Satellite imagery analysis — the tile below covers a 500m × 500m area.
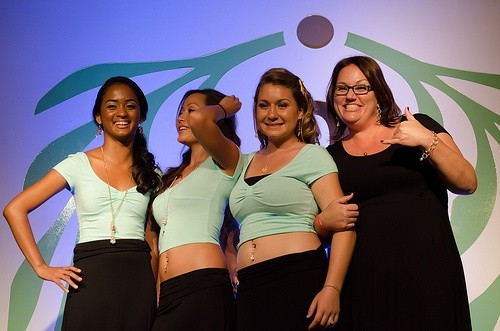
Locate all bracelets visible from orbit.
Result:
[218,104,227,118]
[316,214,327,233]
[323,283,341,294]
[420,131,439,161]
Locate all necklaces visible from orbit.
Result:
[262,138,298,174]
[100,146,132,244]
[162,155,205,236]
[350,127,379,156]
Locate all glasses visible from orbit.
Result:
[333,84,373,96]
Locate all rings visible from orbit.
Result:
[328,318,333,321]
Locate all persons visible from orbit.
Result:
[145,89,243,331]
[3,76,162,331]
[225,67,359,331]
[313,56,477,331]
